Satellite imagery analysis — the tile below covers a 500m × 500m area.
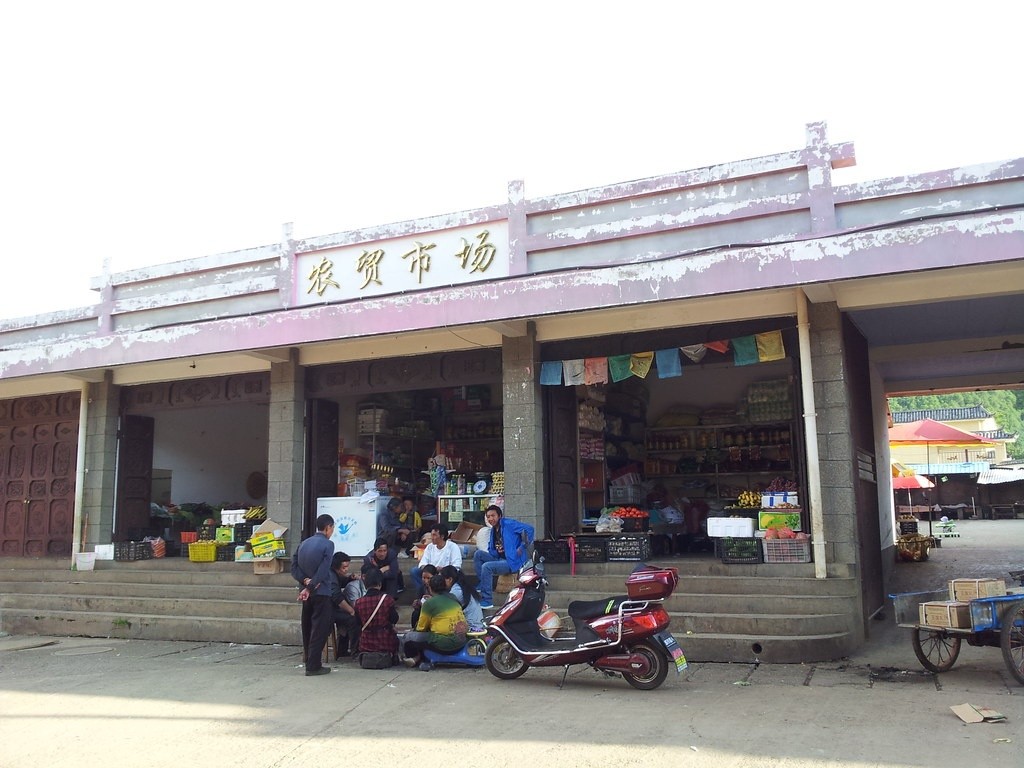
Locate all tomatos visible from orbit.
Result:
[609,506,649,529]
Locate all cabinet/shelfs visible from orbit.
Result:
[639,420,798,501]
[550,383,646,536]
[441,409,503,472]
[354,403,436,507]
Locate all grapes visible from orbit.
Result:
[764,477,797,491]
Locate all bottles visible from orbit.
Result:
[449,474,466,494]
[698,428,714,448]
[466,483,474,494]
[647,429,691,450]
[718,427,790,446]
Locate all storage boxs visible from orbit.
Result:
[896,505,929,512]
[918,578,1024,628]
[95,502,290,574]
[624,566,679,601]
[533,459,677,563]
[337,409,435,497]
[495,572,518,593]
[409,520,485,560]
[707,491,812,564]
[900,522,918,534]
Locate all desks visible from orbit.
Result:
[932,505,983,520]
[986,504,1024,519]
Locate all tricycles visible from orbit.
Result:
[888,569,1024,685]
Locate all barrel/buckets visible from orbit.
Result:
[75,553,95,571]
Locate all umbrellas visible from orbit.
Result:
[893,475,935,516]
[888,418,996,536]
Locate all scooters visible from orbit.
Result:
[480,549,690,691]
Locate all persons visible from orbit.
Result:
[411,565,439,629]
[377,497,414,559]
[399,496,422,531]
[473,505,534,609]
[410,524,463,591]
[364,538,399,594]
[343,563,375,609]
[441,565,484,631]
[290,514,335,676]
[355,567,401,665]
[401,575,468,668]
[330,552,361,662]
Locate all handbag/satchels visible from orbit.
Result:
[595,513,624,534]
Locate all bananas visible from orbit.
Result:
[737,490,761,508]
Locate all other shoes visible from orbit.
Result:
[401,654,416,667]
[396,552,409,559]
[337,651,351,658]
[353,656,359,663]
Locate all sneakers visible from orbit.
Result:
[479,602,493,609]
[475,586,482,592]
[306,667,331,676]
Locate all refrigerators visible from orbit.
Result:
[315,497,395,557]
